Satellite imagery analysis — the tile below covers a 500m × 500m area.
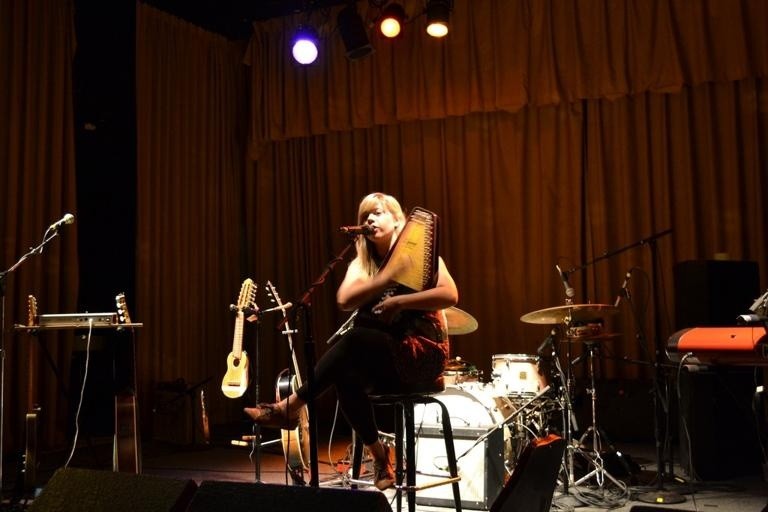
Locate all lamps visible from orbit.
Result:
[289,0,454,67]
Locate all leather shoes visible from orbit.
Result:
[371,442,395,491]
[242,402,300,431]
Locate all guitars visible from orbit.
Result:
[221,278,260,398]
[264,279,311,472]
[113,293,143,473]
[22,296,41,500]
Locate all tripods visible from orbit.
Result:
[561,335,632,496]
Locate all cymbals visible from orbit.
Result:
[521,304,619,325]
[445,306,478,336]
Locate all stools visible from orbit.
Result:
[351,394,461,512]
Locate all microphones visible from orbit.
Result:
[615,271,631,308]
[50,214,74,229]
[336,225,374,234]
[556,265,575,297]
[445,467,460,472]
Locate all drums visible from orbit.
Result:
[399,382,524,512]
[492,355,547,400]
[443,359,479,385]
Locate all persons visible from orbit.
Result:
[239,192,459,492]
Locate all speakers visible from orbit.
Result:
[29,467,197,512]
[413,423,503,510]
[184,480,394,512]
